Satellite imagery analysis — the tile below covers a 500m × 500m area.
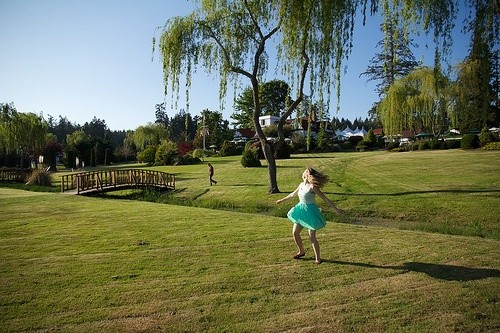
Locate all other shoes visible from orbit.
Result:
[315,259,323,264]
[294,252,306,259]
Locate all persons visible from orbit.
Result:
[276,167,345,264]
[207,162,218,186]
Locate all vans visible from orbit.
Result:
[382,134,410,146]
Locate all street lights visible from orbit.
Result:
[37,155,44,175]
